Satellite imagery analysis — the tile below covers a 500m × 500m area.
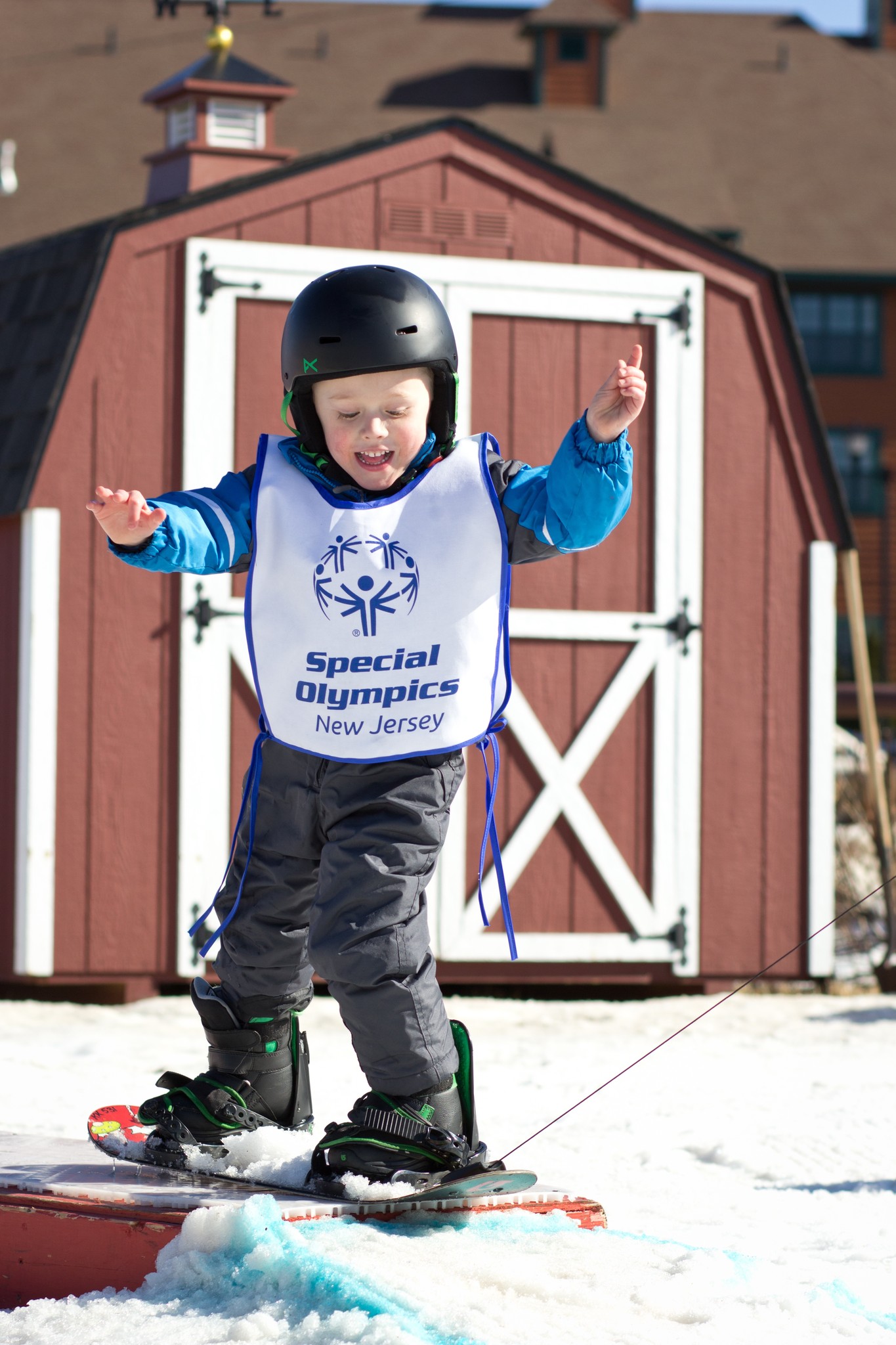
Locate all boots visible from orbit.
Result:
[156,977,313,1139]
[323,1020,477,1175]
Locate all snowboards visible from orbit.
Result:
[86,1106,538,1209]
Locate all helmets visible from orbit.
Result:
[281,264,458,469]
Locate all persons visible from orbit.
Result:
[83,263,652,1190]
